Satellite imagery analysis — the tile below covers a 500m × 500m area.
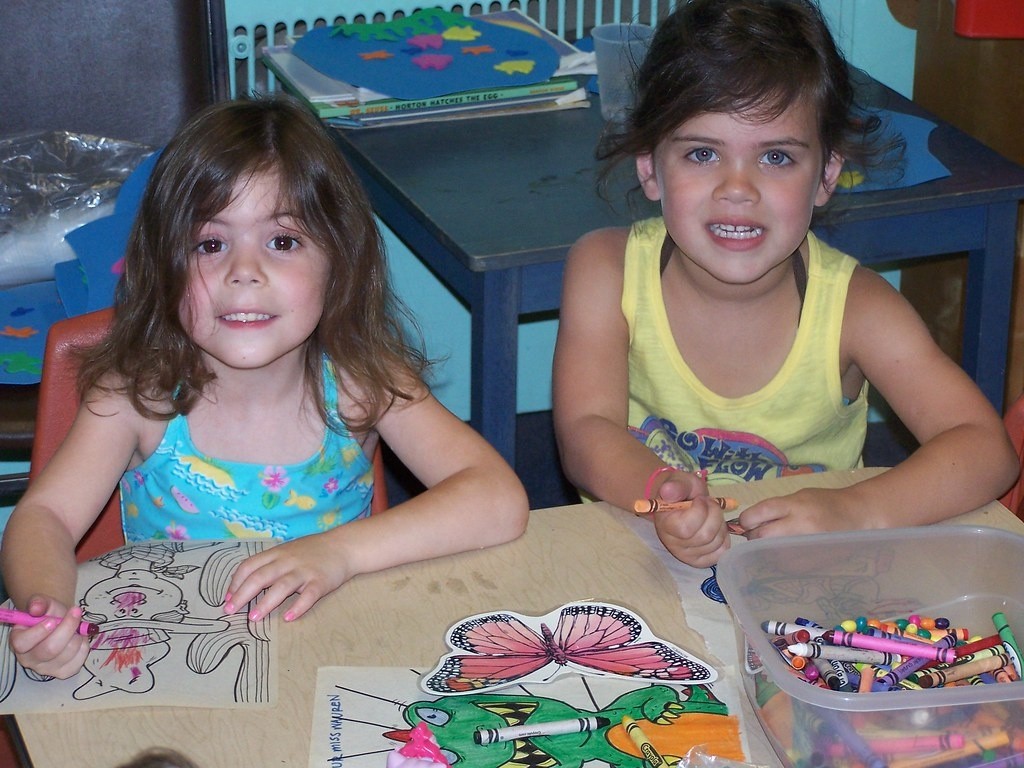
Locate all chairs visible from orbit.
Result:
[26,304,390,567]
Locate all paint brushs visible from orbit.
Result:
[634,496,739,513]
[757,609,1024,767]
[621,714,673,768]
[473,714,611,746]
[0,607,100,637]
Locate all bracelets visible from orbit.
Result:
[643,465,708,518]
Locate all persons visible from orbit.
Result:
[0,88,528,680]
[549,0,1024,570]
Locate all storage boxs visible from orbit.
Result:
[716,526,1023,768]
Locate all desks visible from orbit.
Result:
[1,470,1023,768]
[291,37,1024,504]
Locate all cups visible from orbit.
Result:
[590,22,656,123]
